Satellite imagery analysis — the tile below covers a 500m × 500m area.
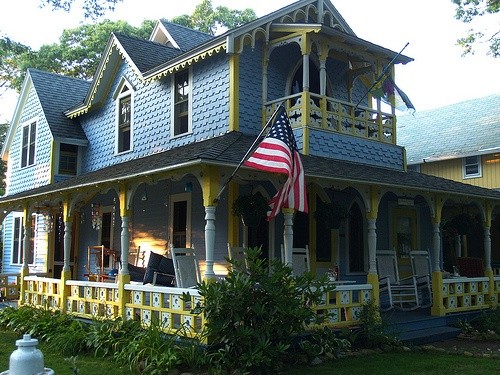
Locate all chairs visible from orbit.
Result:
[226,243,250,280]
[409,248,433,309]
[169,242,204,292]
[280,245,312,280]
[375,247,423,312]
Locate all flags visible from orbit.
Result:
[243,105,309,222]
